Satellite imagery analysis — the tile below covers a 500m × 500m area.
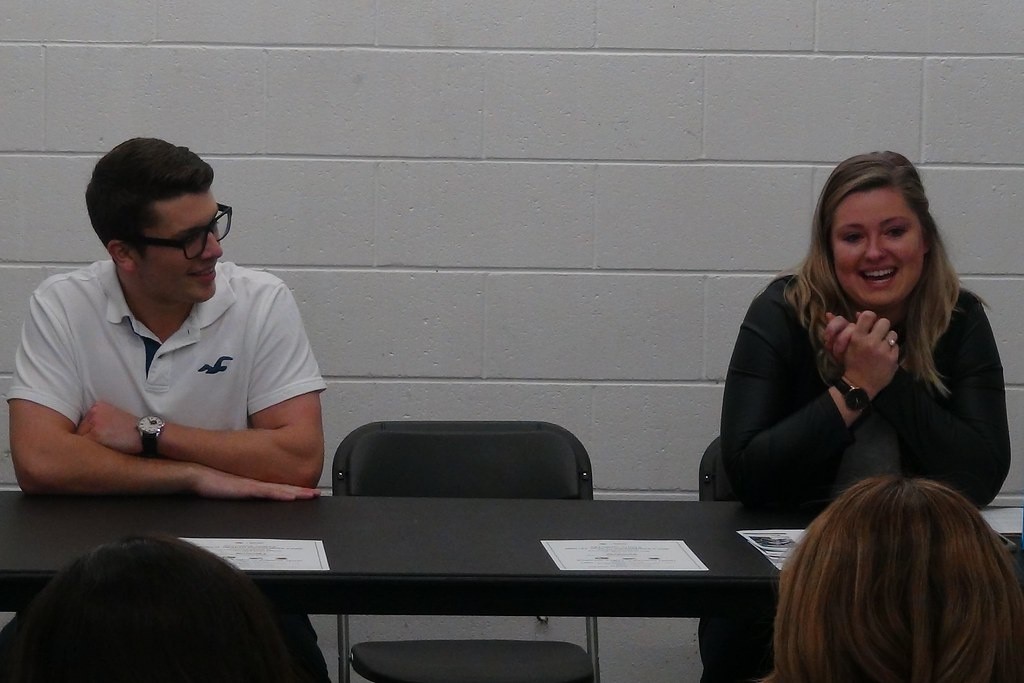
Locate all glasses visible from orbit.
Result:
[114,202,233,260]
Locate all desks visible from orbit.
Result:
[0,488,1024,683]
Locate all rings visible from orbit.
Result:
[885,336,896,347]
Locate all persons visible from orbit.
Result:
[757,476,1024,683]
[5,136,329,499]
[0,533,299,683]
[721,150,1012,505]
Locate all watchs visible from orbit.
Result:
[834,378,874,420]
[137,415,165,456]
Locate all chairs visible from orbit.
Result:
[334,420,600,683]
